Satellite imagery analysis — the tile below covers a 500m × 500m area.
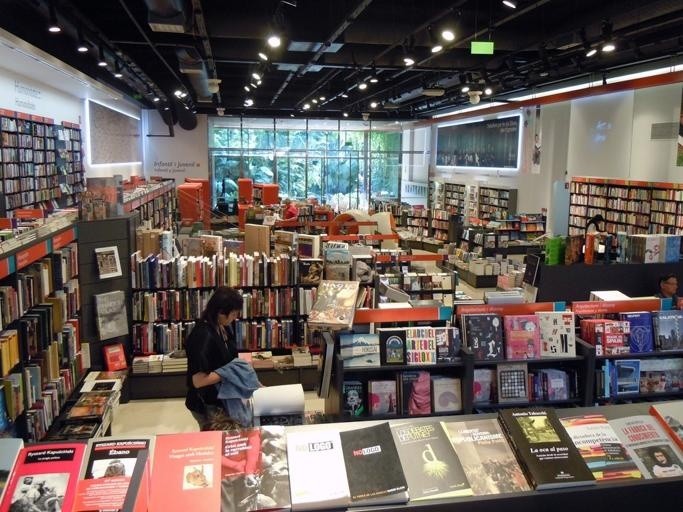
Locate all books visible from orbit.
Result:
[1,181,683,512]
[1,118,84,221]
